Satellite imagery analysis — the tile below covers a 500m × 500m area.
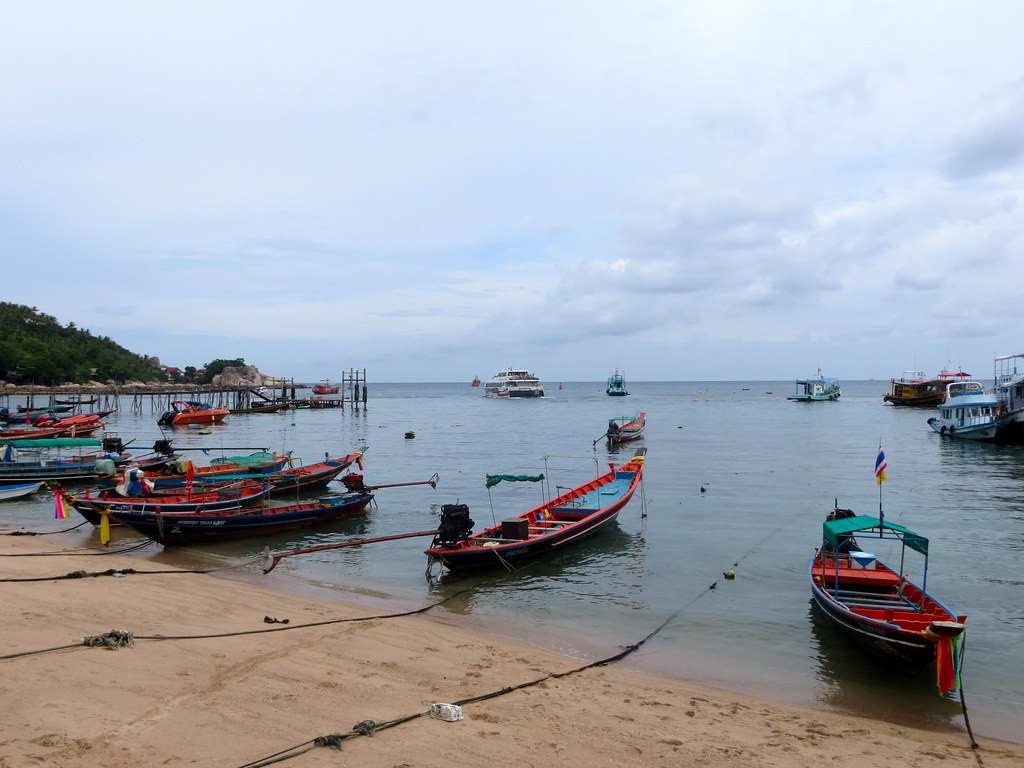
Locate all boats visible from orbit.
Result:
[809,432,966,696]
[199,428,212,435]
[926,351,1024,442]
[472,375,481,386]
[48,420,374,547]
[606,368,629,397]
[189,424,202,429]
[605,411,647,443]
[0,481,45,502]
[424,446,648,571]
[257,385,268,394]
[785,367,841,402]
[881,349,971,406]
[157,400,230,424]
[0,398,136,483]
[484,363,545,397]
[229,403,287,413]
[311,376,339,394]
[559,382,563,390]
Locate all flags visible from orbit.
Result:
[874,445,887,485]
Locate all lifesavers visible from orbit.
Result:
[927,417,936,424]
[808,392,840,402]
[884,397,887,402]
[941,425,955,433]
